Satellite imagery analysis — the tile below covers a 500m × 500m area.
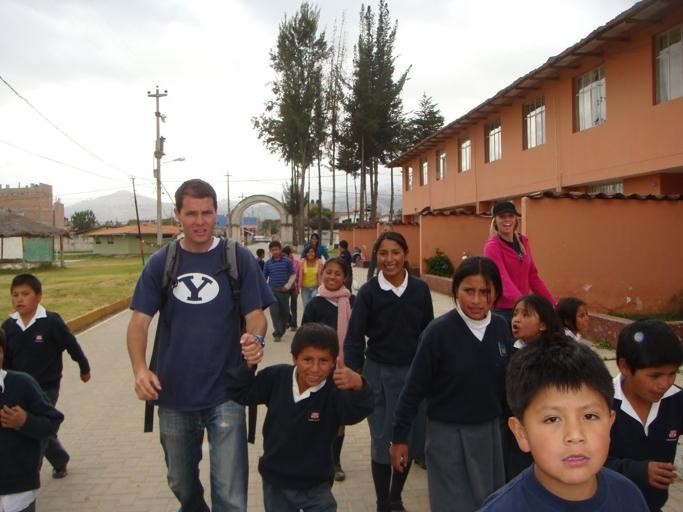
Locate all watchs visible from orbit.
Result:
[254,333,266,350]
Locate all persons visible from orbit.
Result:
[0,273,91,479]
[126,177,268,512]
[553,296,590,336]
[388,255,514,510]
[479,338,651,511]
[483,201,556,319]
[253,232,357,342]
[601,320,683,511]
[299,255,367,482]
[499,293,565,484]
[0,328,63,512]
[226,321,374,511]
[343,232,434,510]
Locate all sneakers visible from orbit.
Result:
[52,463,68,478]
[333,464,345,481]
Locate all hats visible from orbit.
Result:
[493,201,523,218]
